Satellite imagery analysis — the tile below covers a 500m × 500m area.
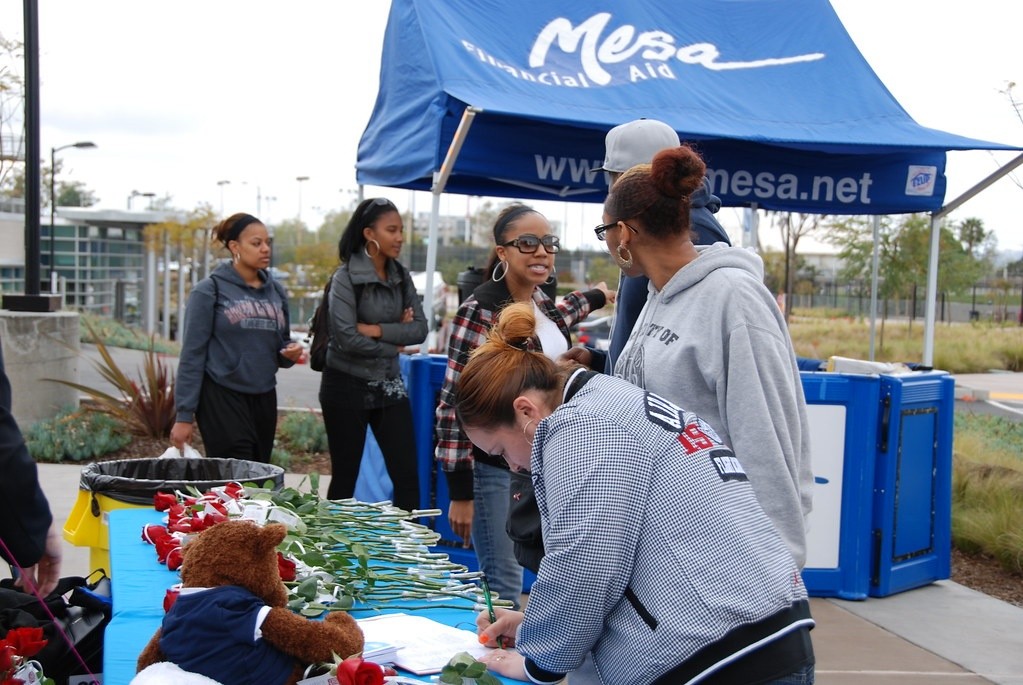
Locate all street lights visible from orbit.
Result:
[218,174,311,337]
[50,141,99,296]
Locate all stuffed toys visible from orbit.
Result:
[136,519,365,685]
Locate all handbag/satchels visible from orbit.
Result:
[51,575,112,672]
[159,442,202,458]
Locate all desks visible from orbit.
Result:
[104,503,539,685]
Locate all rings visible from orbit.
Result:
[496,656,503,661]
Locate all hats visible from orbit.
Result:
[589,117,681,174]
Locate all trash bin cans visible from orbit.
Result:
[62,459,286,585]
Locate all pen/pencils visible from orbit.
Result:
[478,572,502,649]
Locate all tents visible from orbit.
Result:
[357,1,1023,368]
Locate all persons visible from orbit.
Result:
[436,117,813,610]
[454,302,816,685]
[318,198,428,525]
[170,213,303,462]
[0,355,63,598]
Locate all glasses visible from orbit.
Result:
[362,198,396,216]
[594,221,638,240]
[501,234,560,255]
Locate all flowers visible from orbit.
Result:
[140,473,514,685]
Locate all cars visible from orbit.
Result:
[168,257,316,286]
[411,270,450,320]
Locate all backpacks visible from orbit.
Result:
[310,261,407,372]
